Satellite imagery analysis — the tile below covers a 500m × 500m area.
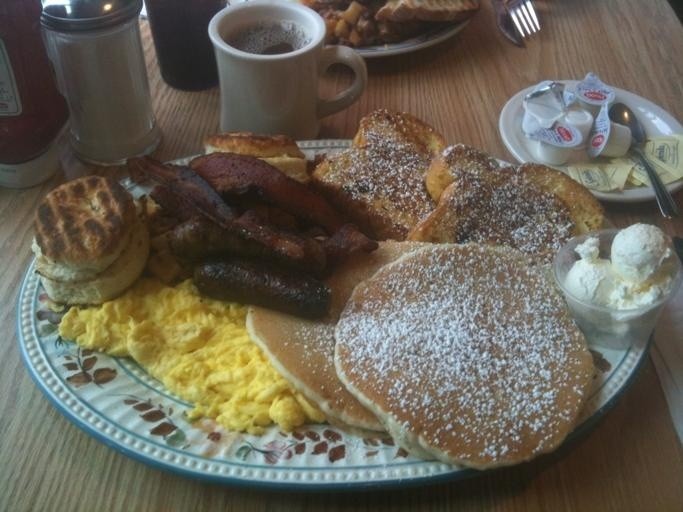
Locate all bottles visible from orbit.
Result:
[0,0,68,164]
[144,0,223,93]
[39,0,164,166]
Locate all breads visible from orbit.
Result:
[31,173,153,306]
[378,0,475,23]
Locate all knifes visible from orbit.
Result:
[489,1,525,51]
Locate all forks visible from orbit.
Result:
[508,1,542,34]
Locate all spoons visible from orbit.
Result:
[608,102,676,223]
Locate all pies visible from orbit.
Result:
[206,108,612,472]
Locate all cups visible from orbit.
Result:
[205,0,367,137]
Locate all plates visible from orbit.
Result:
[496,80,681,206]
[347,8,486,58]
[15,138,653,492]
